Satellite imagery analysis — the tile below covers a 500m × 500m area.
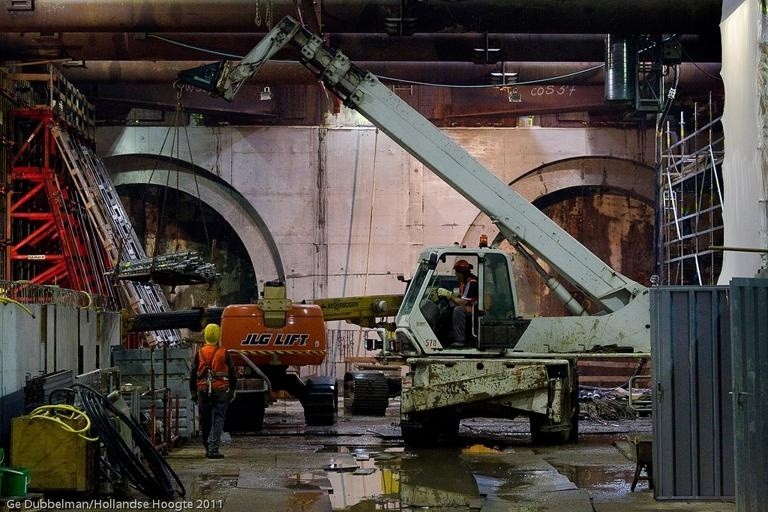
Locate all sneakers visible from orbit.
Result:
[205,451,225,459]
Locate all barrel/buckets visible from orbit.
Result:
[0,467,31,496]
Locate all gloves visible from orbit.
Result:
[438,287,454,299]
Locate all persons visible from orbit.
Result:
[436,260,478,346]
[190,323,236,460]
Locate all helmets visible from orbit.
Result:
[204,323,219,345]
[453,260,469,270]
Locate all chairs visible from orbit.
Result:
[482,293,496,310]
